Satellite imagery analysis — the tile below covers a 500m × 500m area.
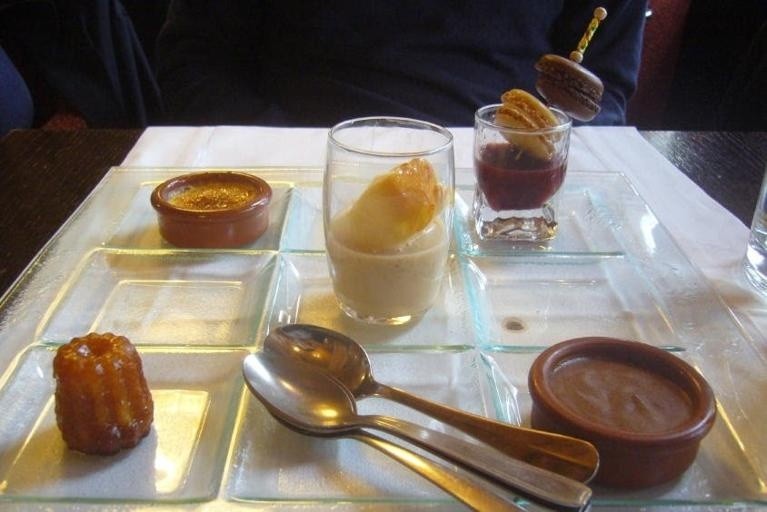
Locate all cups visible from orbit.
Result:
[475,102,573,240]
[323,116,455,324]
[744,166,767,296]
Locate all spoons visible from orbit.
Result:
[266,326,598,487]
[240,354,595,512]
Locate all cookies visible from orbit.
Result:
[534,51,605,123]
[496,89,560,163]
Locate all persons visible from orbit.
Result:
[153,0,648,126]
